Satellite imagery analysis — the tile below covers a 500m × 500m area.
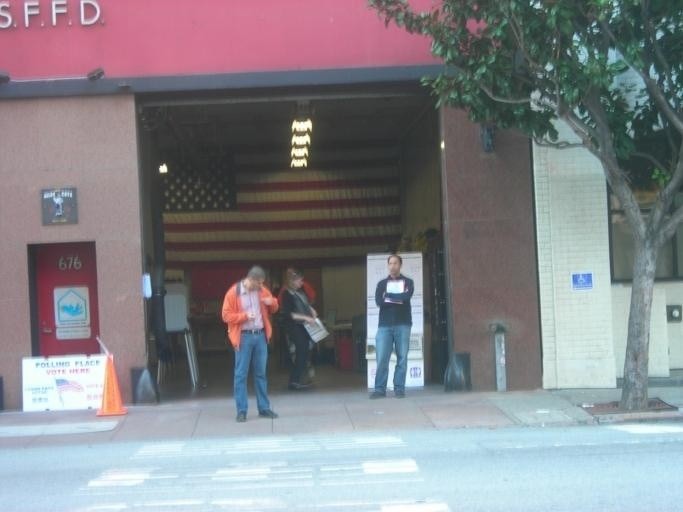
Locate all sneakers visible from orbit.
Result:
[368,391,405,400]
[286,381,311,391]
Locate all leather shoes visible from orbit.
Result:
[258,409,278,419]
[236,411,246,422]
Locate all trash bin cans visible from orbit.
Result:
[334,323,354,371]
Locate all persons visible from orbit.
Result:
[275,265,321,389]
[368,254,414,400]
[221,266,282,422]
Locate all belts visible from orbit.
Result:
[239,327,264,335]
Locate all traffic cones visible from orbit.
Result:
[96,355,128,416]
